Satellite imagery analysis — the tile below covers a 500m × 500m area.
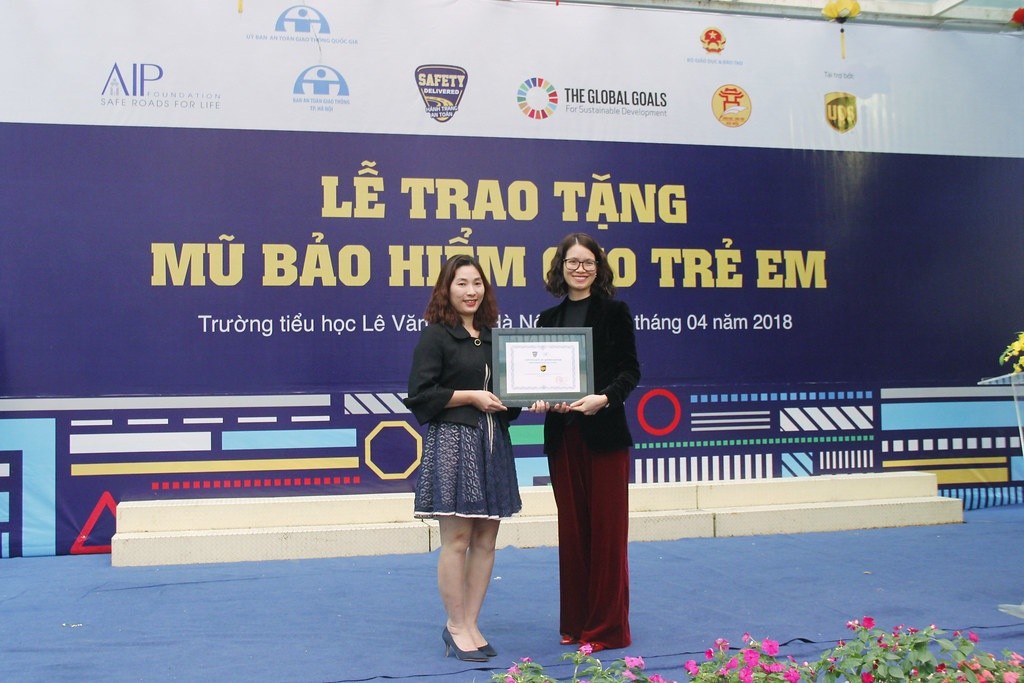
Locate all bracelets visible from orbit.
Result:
[605,403,610,409]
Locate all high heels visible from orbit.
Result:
[476,643,498,658]
[441,627,489,663]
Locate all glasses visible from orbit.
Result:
[563,257,599,272]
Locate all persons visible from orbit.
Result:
[403,254,550,661]
[537,233,641,655]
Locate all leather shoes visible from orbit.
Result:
[578,640,609,653]
[560,633,578,645]
